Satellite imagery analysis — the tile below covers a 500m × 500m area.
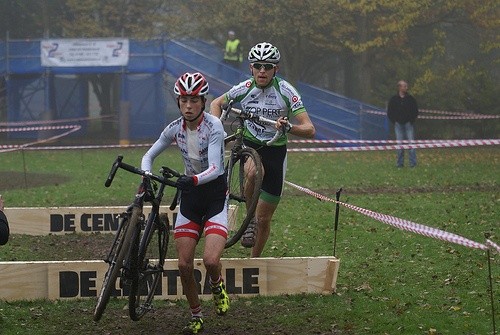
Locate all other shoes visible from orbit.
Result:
[240,215,258,248]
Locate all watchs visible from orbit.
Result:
[288,123,293,133]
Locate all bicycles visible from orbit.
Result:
[219,100,290,249]
[93,155,193,322]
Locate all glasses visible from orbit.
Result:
[253,63,276,70]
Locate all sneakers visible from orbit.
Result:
[180,315,205,334]
[211,279,230,315]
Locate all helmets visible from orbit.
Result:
[247,42,280,63]
[173,73,208,97]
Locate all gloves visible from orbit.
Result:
[177,175,194,192]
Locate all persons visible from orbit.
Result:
[210,41,314,257]
[387,79,418,169]
[0,193,10,246]
[142,72,232,335]
